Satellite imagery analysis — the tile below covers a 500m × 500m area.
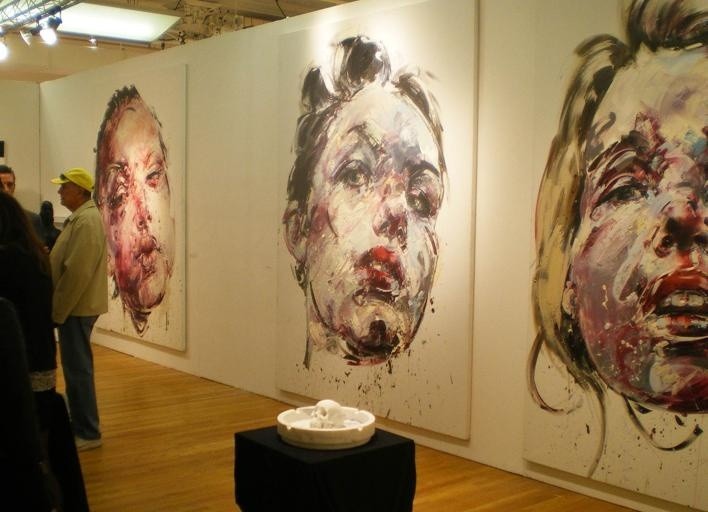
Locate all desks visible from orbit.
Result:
[232,424,416,512]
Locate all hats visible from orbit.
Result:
[48,166,96,194]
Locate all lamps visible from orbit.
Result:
[19,6,63,48]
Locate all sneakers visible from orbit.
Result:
[73,431,103,455]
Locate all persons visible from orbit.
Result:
[0,164,92,512]
[526,0,708,416]
[283,34,450,371]
[90,84,176,338]
[48,167,108,452]
